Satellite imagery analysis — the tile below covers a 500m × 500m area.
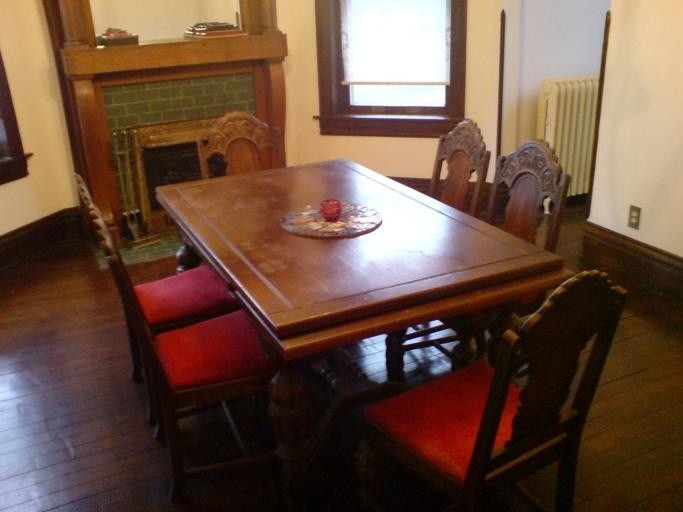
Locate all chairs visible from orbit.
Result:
[488,139,571,255]
[72,171,280,503]
[196,113,276,175]
[428,116,491,218]
[373,270,632,509]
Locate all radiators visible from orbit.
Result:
[536,75,602,210]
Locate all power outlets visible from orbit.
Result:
[628,202,641,229]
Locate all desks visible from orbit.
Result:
[151,160,572,474]
